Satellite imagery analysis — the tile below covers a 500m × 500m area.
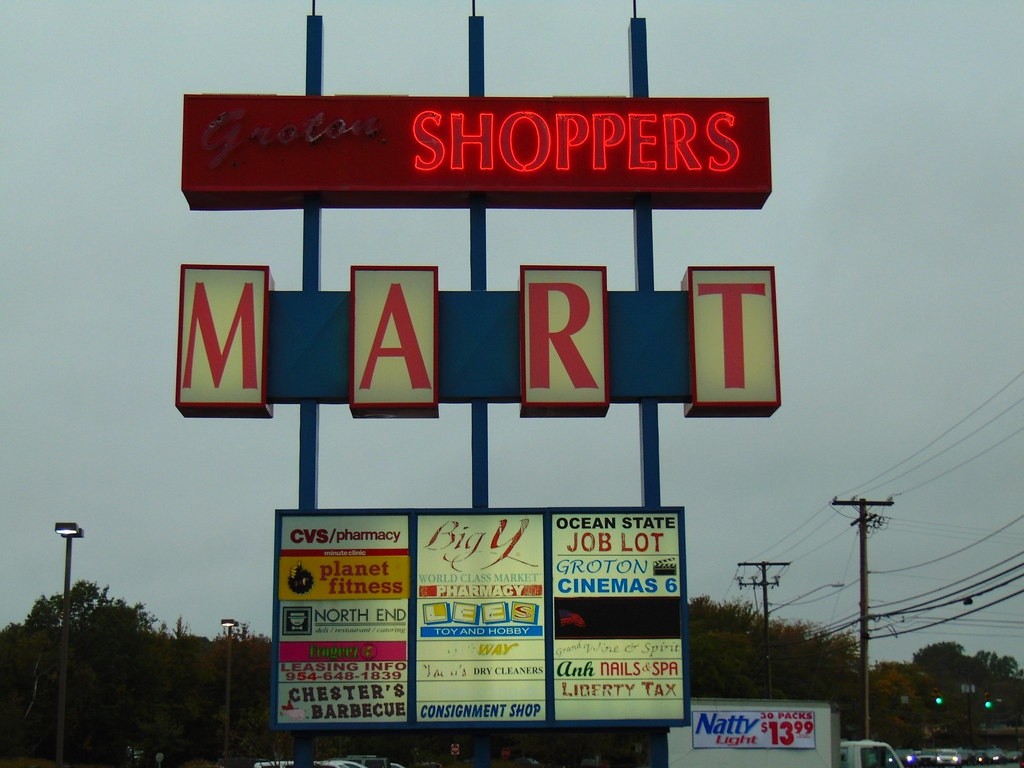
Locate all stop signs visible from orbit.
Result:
[502,747,510,759]
[451,744,459,756]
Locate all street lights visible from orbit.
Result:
[221,617,240,759]
[763,582,845,700]
[54,524,84,768]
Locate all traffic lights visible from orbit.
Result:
[935,695,943,706]
[986,700,991,709]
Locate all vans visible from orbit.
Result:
[840,740,905,767]
[581,753,610,768]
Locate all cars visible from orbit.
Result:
[514,757,539,766]
[894,746,1023,768]
[256,755,405,768]
